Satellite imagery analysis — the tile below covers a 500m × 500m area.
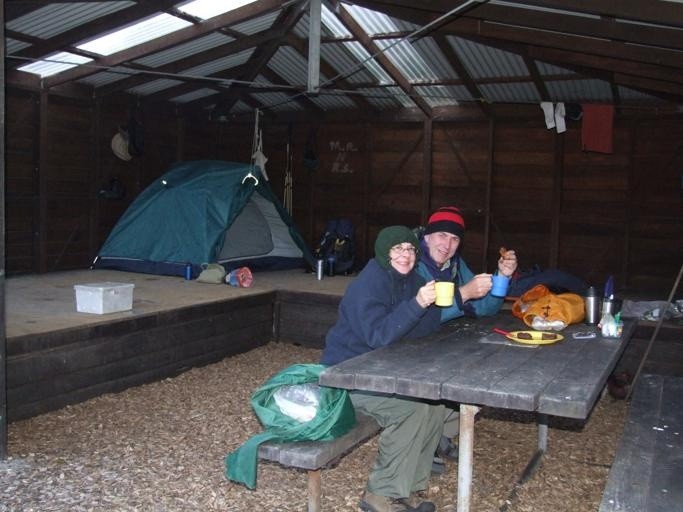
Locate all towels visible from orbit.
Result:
[580,104,615,155]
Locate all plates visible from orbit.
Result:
[504,326,563,347]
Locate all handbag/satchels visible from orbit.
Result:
[512,283,585,328]
[196,263,253,288]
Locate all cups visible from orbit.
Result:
[328,257,336,277]
[185,262,193,280]
[584,285,600,324]
[316,260,325,280]
[601,286,616,323]
[490,274,512,299]
[435,282,456,307]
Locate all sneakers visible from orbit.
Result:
[359,489,435,512]
[432,435,459,474]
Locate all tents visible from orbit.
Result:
[85,156,316,282]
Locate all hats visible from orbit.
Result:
[424,207,465,242]
[375,225,421,268]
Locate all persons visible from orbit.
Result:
[316,225,450,512]
[409,204,521,477]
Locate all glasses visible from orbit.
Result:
[388,246,417,256]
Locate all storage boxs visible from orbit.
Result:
[74,281,135,315]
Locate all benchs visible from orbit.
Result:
[257,412,382,512]
[598,372,683,512]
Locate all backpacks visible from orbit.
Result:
[313,218,356,275]
